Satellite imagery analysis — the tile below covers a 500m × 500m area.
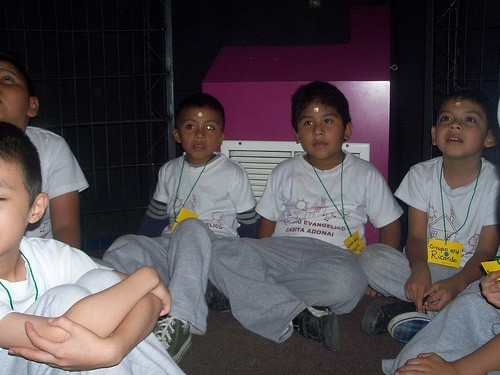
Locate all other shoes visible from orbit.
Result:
[386,311,431,343]
[360,293,416,337]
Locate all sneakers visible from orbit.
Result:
[292,304,341,353]
[202,278,233,312]
[153,312,195,364]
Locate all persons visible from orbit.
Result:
[0,121,187,375]
[358,89,500,343]
[382,270,500,375]
[205,81,404,351]
[0,58,90,249]
[102,93,262,364]
